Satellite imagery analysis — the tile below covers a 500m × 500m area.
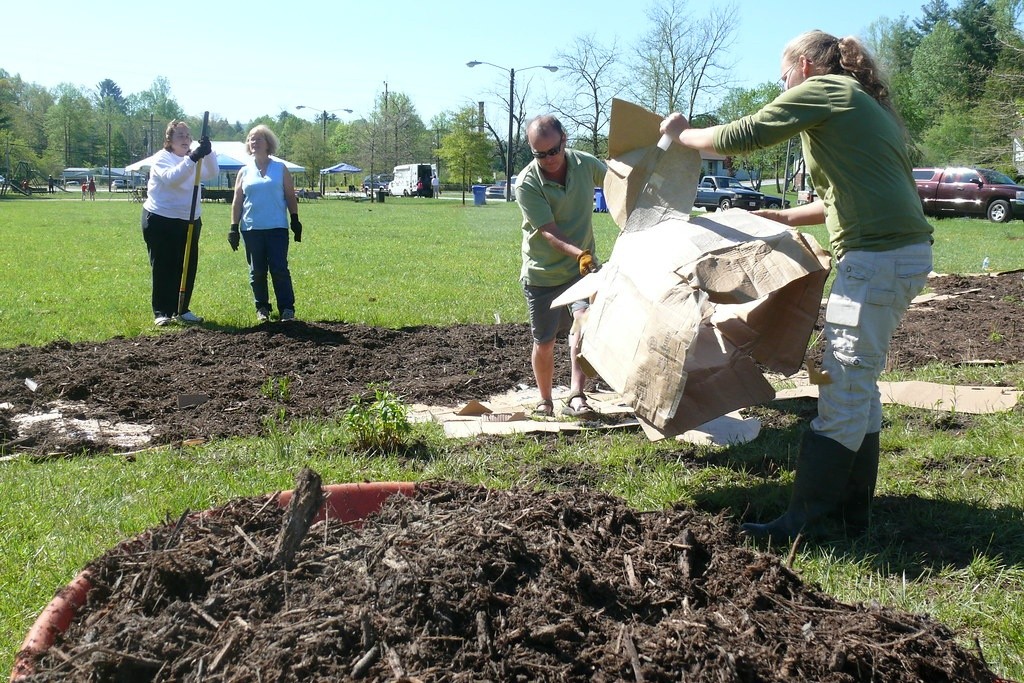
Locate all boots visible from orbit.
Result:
[829,431,879,536]
[740,429,857,545]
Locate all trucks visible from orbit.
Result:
[112,180,135,188]
[388,164,435,198]
[363,174,394,196]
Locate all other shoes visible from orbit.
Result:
[154,316,171,327]
[171,310,204,325]
[280,309,294,322]
[256,308,271,322]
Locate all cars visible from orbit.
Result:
[485,177,517,201]
[0,175,5,186]
[912,166,1024,223]
[761,195,790,208]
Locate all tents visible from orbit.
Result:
[319,163,362,200]
[124,141,305,202]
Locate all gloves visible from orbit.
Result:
[290,213,302,242]
[198,135,212,156]
[228,224,240,251]
[578,248,596,276]
[189,145,209,162]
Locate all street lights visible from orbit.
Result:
[296,106,353,194]
[466,61,558,201]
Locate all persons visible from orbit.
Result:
[227,124,302,320]
[431,175,439,199]
[140,187,147,198]
[136,187,139,194]
[659,27,934,541]
[88,178,97,201]
[142,119,218,325]
[48,175,53,193]
[514,114,607,416]
[416,177,424,198]
[81,182,87,201]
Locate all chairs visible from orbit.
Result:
[129,190,145,203]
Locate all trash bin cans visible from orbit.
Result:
[375,191,385,202]
[594,188,609,213]
[472,185,487,205]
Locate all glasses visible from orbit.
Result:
[531,134,563,159]
[776,60,812,92]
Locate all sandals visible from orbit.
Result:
[561,392,595,418]
[531,399,555,419]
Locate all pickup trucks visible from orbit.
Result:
[694,175,765,212]
